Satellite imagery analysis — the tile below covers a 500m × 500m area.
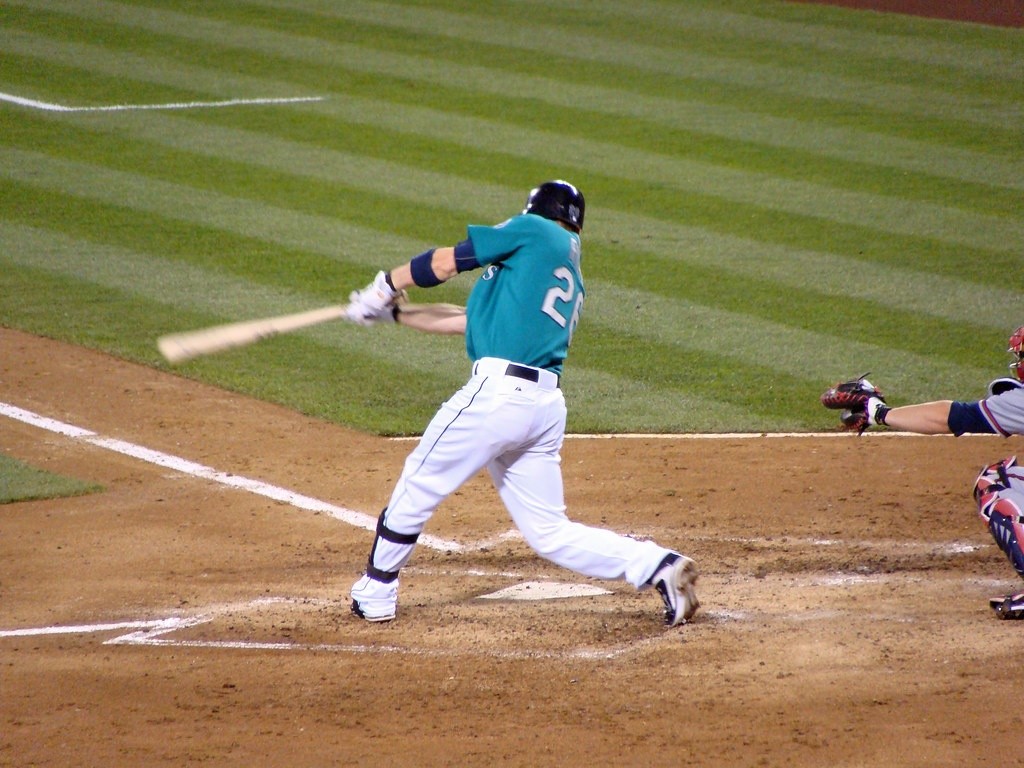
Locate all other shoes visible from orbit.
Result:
[996,600,1024,620]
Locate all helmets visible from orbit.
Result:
[1007,326,1024,383]
[520,179,585,232]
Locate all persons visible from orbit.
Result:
[821,324,1024,618]
[347,179,699,630]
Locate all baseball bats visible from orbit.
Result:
[155,287,411,364]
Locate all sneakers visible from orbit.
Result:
[350,573,398,622]
[646,552,699,628]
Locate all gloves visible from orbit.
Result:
[342,271,397,326]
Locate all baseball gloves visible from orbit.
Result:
[820,374,889,431]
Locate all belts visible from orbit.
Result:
[475,362,560,388]
